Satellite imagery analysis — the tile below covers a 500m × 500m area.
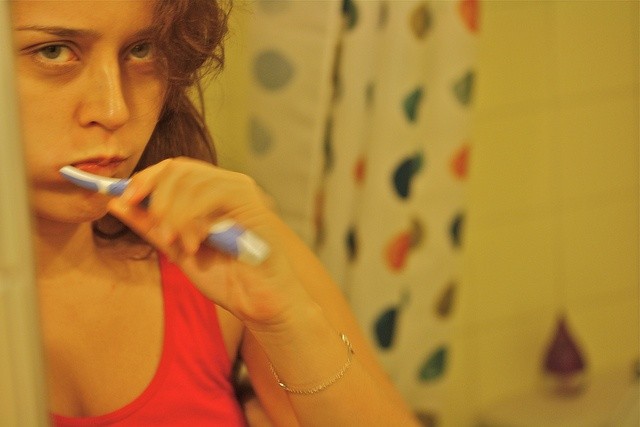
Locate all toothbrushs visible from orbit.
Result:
[51,162,275,277]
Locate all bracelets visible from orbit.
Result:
[264,330,356,398]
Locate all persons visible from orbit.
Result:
[1,1,419,427]
